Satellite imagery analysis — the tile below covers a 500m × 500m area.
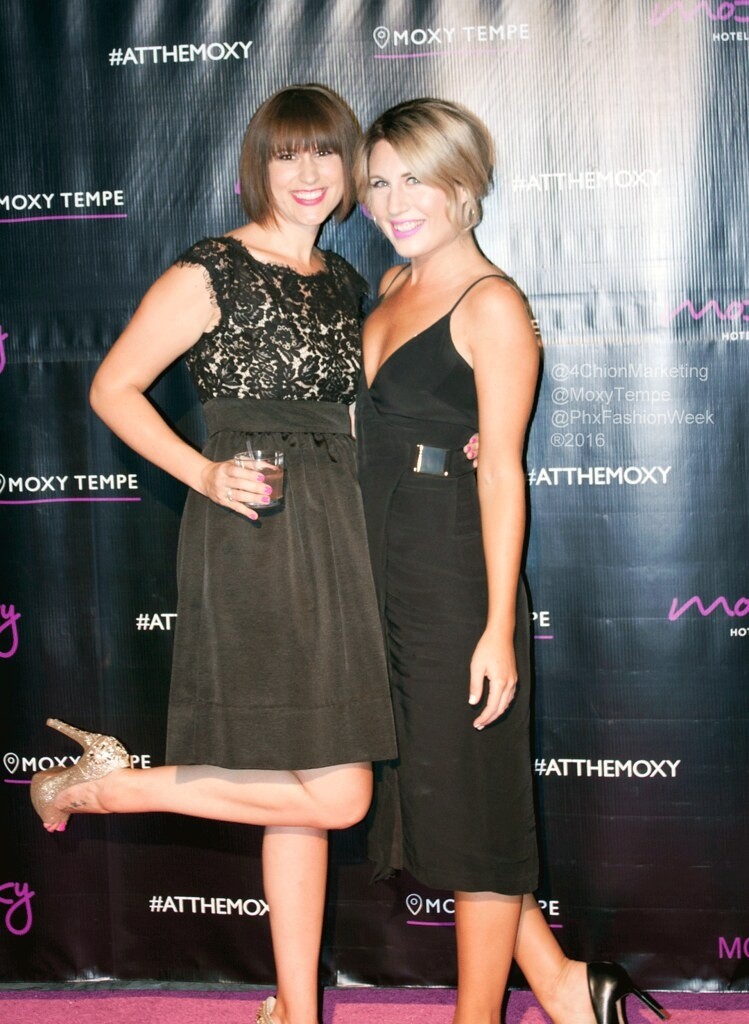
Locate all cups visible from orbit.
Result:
[234,450,284,509]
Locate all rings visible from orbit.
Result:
[228,488,233,501]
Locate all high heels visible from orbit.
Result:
[256,996,277,1024]
[30,718,129,824]
[584,961,669,1024]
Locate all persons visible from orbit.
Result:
[355,98,675,1024]
[30,82,479,1024]
